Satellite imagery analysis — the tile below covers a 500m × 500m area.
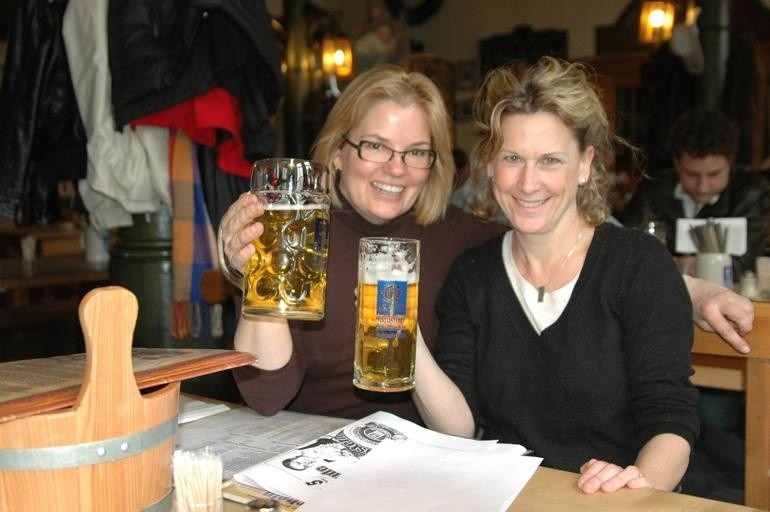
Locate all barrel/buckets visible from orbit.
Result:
[108,208,236,404]
[0,285,182,512]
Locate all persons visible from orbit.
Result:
[352,55,702,492]
[214,57,756,436]
[627,105,770,291]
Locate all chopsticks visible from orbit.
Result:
[688,218,729,254]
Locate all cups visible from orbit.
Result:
[216,156,334,322]
[352,234,422,393]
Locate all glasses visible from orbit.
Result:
[339,133,437,172]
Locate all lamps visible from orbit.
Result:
[639,0,676,45]
[314,21,353,81]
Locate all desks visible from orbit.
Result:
[0,215,116,358]
[169,392,770,510]
[690,278,770,511]
